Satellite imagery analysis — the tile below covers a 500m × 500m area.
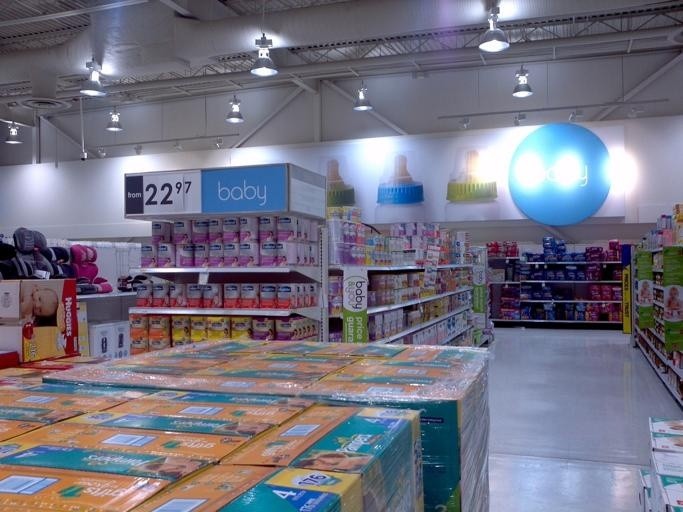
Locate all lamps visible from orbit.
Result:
[513,64,534,99]
[477,7,509,51]
[78,61,105,98]
[106,105,122,132]
[251,3,278,76]
[5,125,22,146]
[353,81,373,111]
[225,93,244,123]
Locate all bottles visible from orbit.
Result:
[444,149,501,221]
[374,153,427,224]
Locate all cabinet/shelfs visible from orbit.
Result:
[2,238,145,359]
[632,243,682,407]
[126,216,329,343]
[323,217,474,344]
[488,257,623,325]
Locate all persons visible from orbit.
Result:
[35,409,79,424]
[224,328,229,338]
[221,421,271,436]
[266,328,274,340]
[665,288,683,319]
[146,457,203,480]
[640,281,654,305]
[149,231,316,268]
[184,326,190,337]
[295,452,370,472]
[20,283,58,323]
[248,328,253,338]
[272,399,314,408]
[291,325,317,340]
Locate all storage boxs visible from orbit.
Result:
[0,342,489,512]
[637,413,683,512]
